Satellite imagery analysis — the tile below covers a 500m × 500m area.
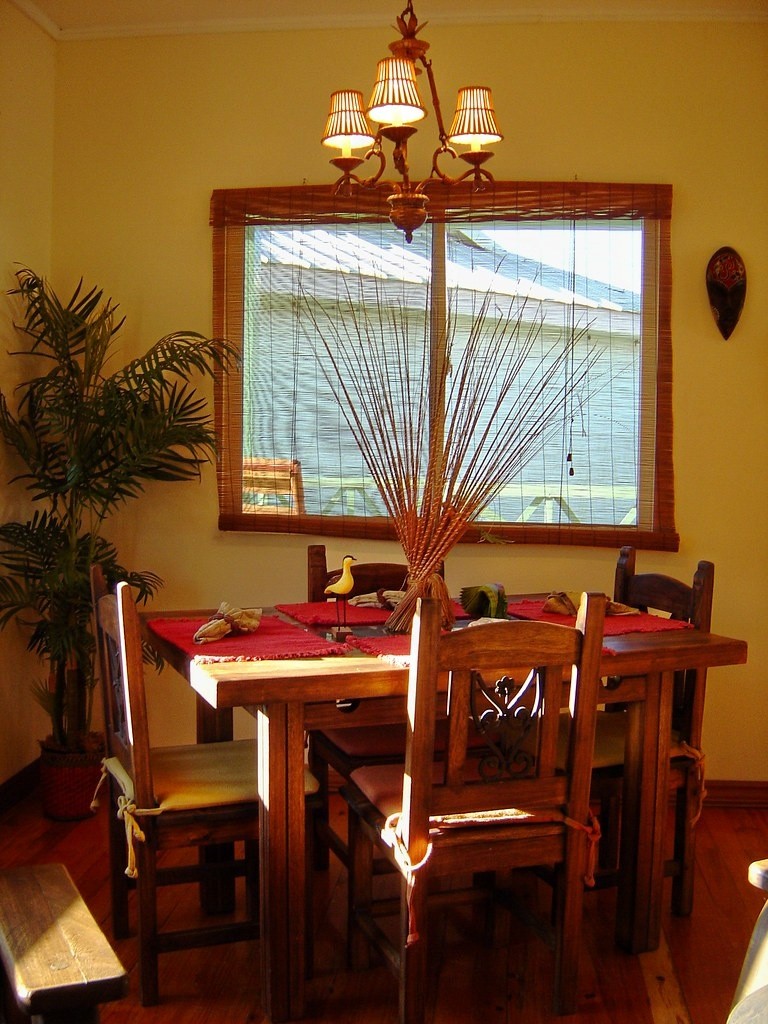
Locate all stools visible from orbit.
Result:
[0,864,130,1024]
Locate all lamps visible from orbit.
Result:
[320,0,504,244]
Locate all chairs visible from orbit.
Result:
[89,564,320,1008]
[507,545,715,915]
[307,543,446,939]
[347,591,607,1024]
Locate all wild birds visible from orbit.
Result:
[324,555,357,632]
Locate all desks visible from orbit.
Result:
[138,593,749,1024]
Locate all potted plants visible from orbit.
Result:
[0,248,242,815]
